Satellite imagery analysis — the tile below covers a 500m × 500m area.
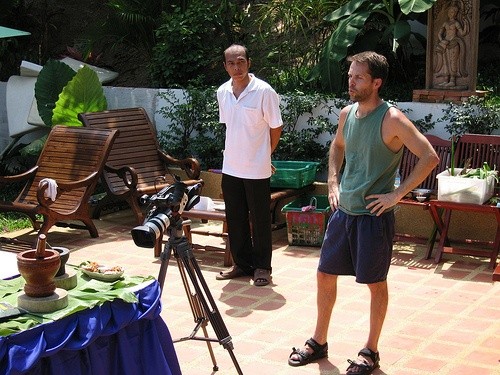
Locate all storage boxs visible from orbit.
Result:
[280,195,331,248]
[270,161,321,189]
[435,168,499,205]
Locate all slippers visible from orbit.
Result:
[216,265,249,280]
[253,267,271,285]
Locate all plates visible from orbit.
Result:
[82,263,125,281]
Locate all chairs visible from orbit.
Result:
[78,107,200,226]
[0,125,120,249]
[394,134,500,271]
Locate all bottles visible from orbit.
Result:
[394,169,401,189]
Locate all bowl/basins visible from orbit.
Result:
[411,188,432,199]
[415,197,426,202]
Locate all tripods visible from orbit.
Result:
[158,219,244,375]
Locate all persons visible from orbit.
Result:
[437,7,467,87]
[217,44,283,287]
[289,52,440,375]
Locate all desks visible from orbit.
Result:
[154,183,316,267]
[0,263,183,375]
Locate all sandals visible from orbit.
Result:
[288,336,328,366]
[346,346,380,375]
